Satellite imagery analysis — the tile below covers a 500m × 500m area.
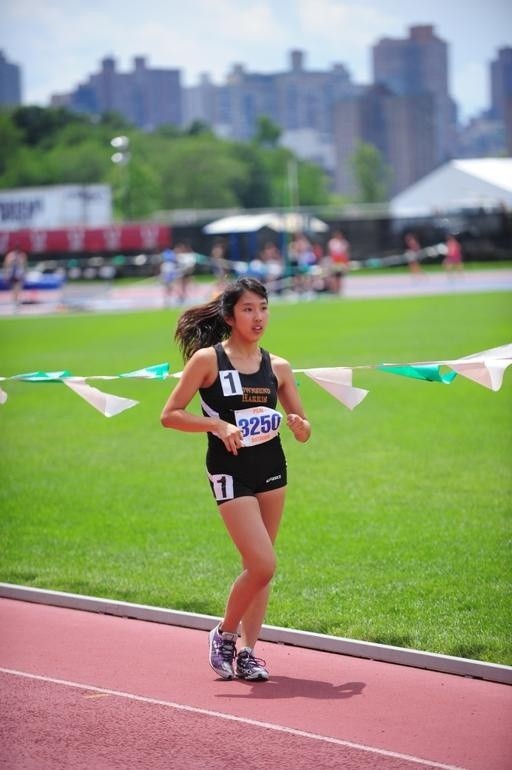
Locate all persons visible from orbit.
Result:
[4,244,27,308]
[404,232,422,274]
[154,225,350,305]
[441,233,464,273]
[157,276,312,683]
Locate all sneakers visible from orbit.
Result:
[209,622,269,681]
